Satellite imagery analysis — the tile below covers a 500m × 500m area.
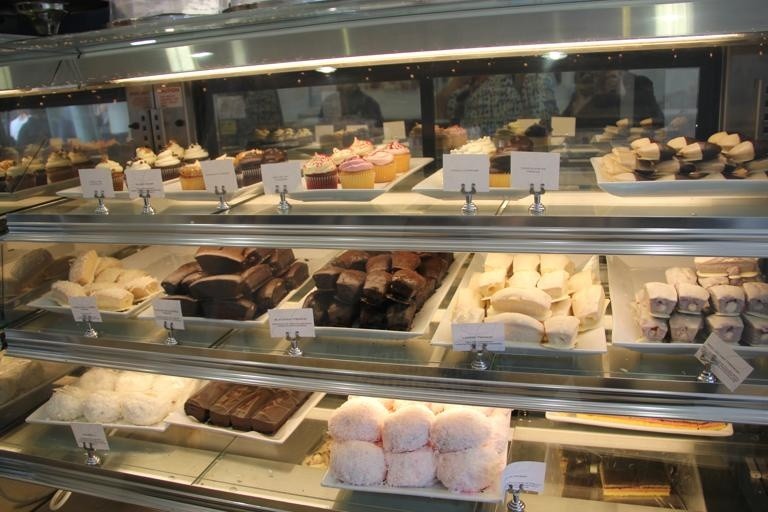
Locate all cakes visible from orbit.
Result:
[330,135,410,189]
[303,151,337,190]
[179,163,205,190]
[602,130,767,182]
[499,116,544,137]
[629,256,767,347]
[250,122,310,142]
[450,128,533,174]
[0,147,89,191]
[0,247,77,298]
[183,143,209,164]
[447,123,466,146]
[216,148,288,188]
[94,160,123,191]
[124,140,184,188]
[595,117,653,143]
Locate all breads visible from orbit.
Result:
[48,249,159,312]
[447,252,604,348]
[183,380,315,437]
[325,400,508,493]
[0,348,44,406]
[301,250,454,331]
[598,457,671,499]
[43,366,193,426]
[153,246,309,320]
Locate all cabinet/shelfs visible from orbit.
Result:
[1,1,768,512]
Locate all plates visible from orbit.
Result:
[319,394,512,506]
[136,248,342,329]
[429,252,608,357]
[410,124,567,192]
[24,378,209,433]
[55,146,210,201]
[0,244,100,308]
[298,252,471,340]
[162,153,305,200]
[605,254,767,356]
[25,244,204,320]
[545,411,733,438]
[162,380,326,445]
[287,156,434,200]
[589,155,768,195]
[0,139,106,200]
[1,352,86,425]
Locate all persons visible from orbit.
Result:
[563,69,666,127]
[17,106,51,145]
[319,81,383,120]
[9,109,32,146]
[435,72,560,131]
